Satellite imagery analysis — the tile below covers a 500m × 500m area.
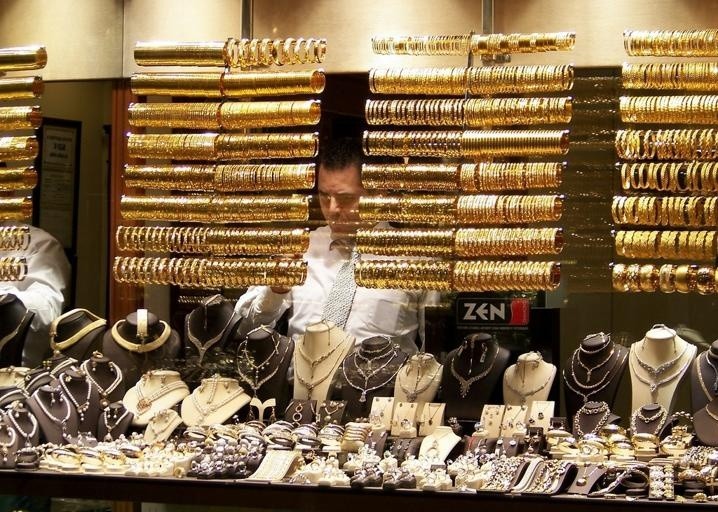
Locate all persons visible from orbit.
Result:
[0,222,73,326]
[230,146,440,359]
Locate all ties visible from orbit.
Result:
[320,251,360,332]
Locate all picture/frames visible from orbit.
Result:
[32,114,83,317]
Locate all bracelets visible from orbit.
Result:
[611,29,718,293]
[0,47,48,281]
